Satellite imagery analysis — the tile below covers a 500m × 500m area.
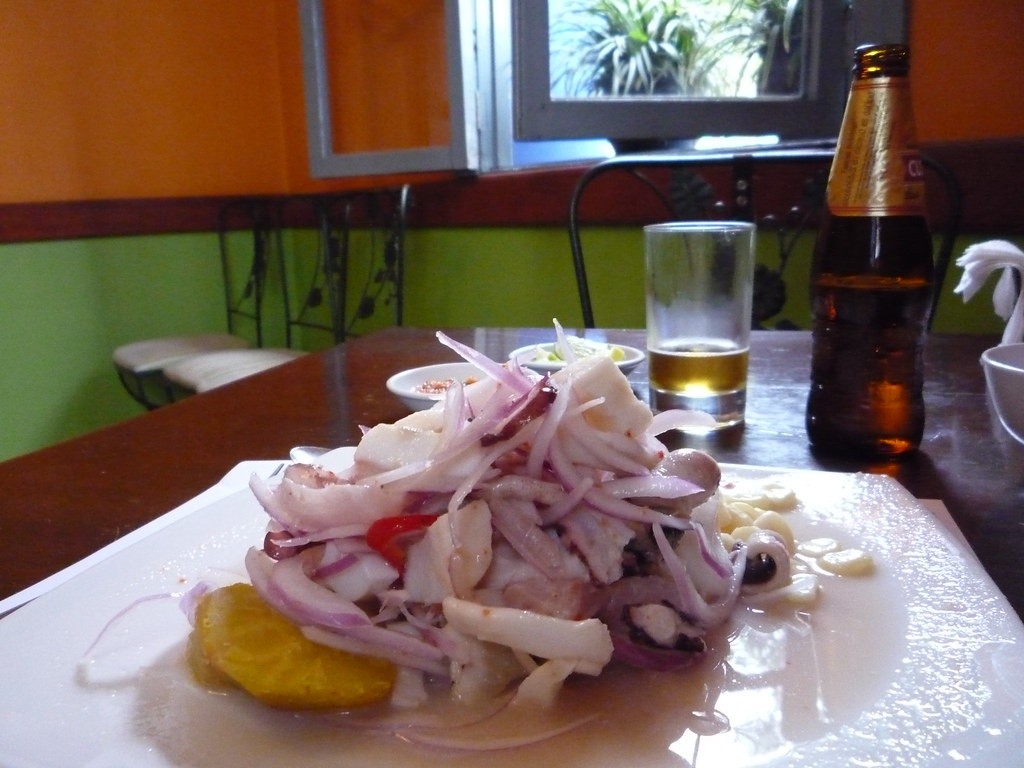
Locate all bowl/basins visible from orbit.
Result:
[981,343,1024,443]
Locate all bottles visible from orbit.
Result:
[806,42,935,455]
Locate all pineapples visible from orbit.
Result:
[192,582,393,708]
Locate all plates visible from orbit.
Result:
[0,461,1024,768]
[385,362,544,412]
[508,341,645,377]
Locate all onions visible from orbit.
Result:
[81,316,751,751]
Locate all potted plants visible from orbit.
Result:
[550,0,735,156]
[700,0,802,96]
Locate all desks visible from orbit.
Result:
[0,323,1024,768]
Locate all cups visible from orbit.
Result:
[644,221,757,429]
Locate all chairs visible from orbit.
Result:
[112,196,274,411]
[160,184,410,406]
[568,148,963,332]
